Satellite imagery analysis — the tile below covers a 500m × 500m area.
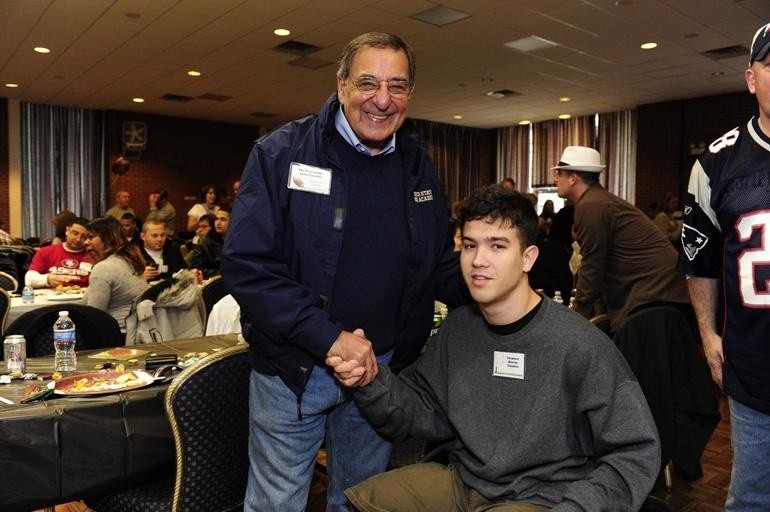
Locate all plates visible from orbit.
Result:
[47,294,82,300]
[54,370,154,395]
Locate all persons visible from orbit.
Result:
[1,174,240,349]
[218,30,467,509]
[324,23,769,512]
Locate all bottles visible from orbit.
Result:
[569,288,577,306]
[552,291,563,302]
[53,310,77,371]
[22,282,34,304]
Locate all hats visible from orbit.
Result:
[747,23,769,68]
[551,144,608,173]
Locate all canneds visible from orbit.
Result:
[3,334,26,375]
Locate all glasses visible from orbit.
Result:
[353,75,410,97]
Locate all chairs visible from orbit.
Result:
[123,279,206,338]
[82,345,251,512]
[4,303,123,356]
[609,310,721,490]
[0,272,19,296]
[0,288,11,338]
[198,276,227,317]
[588,315,612,335]
